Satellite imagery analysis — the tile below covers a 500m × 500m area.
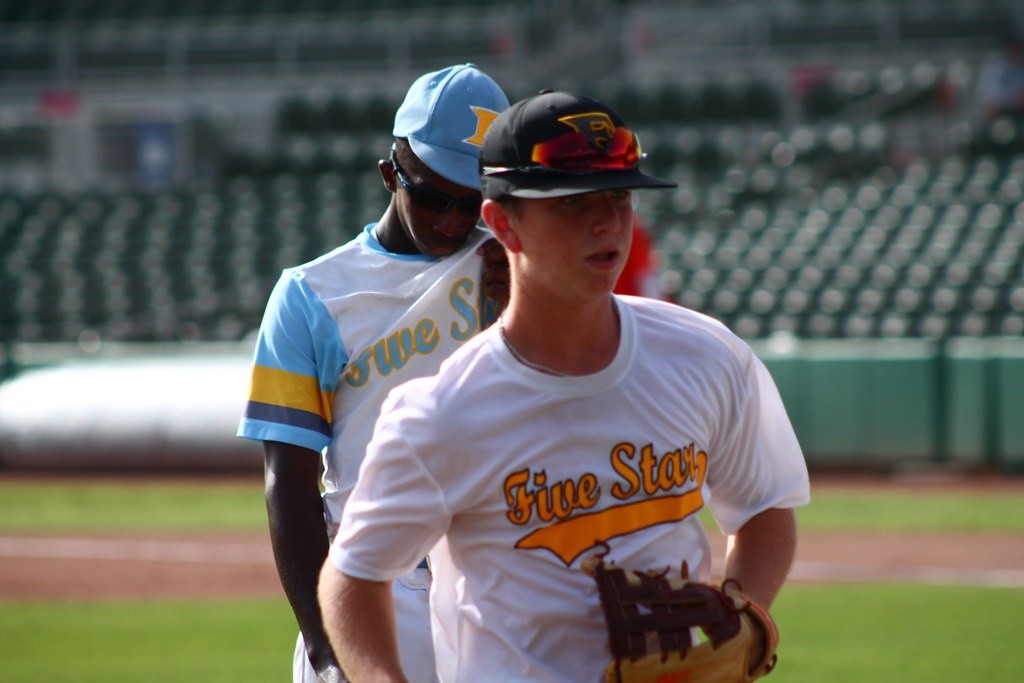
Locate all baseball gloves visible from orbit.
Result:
[581,537,780,682]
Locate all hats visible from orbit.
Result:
[392,62,512,192]
[479,89,677,201]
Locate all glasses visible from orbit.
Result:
[388,149,483,216]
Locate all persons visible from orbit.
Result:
[316,83,811,683]
[236,62,515,683]
